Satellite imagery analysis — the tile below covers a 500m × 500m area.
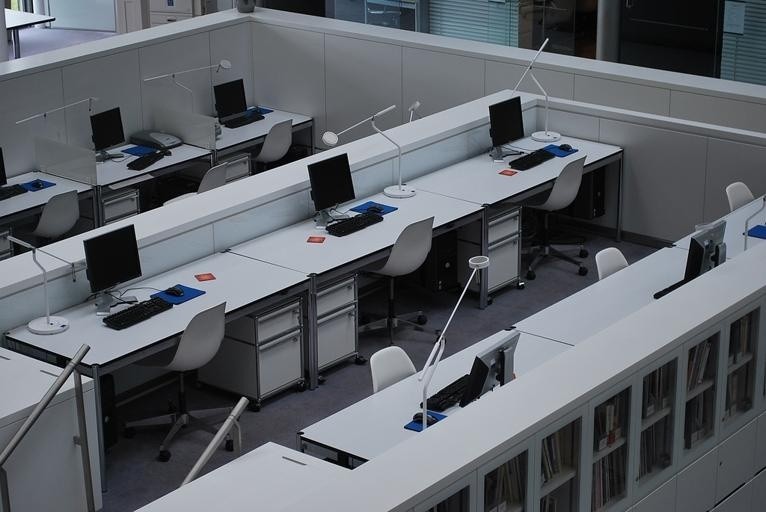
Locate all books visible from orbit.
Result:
[540,478,577,511]
[540,419,576,486]
[685,388,713,449]
[643,362,673,419]
[687,341,710,391]
[430,489,470,511]
[640,418,672,480]
[728,315,749,368]
[593,391,626,452]
[483,456,526,511]
[593,446,626,511]
[724,362,753,417]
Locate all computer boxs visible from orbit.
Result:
[101,374,118,447]
[565,166,605,221]
[410,232,458,309]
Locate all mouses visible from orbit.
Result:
[413,412,438,426]
[413,410,438,429]
[164,287,186,298]
[367,206,384,213]
[31,182,45,189]
[161,148,172,157]
[559,144,573,153]
[560,144,572,152]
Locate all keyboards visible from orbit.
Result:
[325,211,384,237]
[419,374,469,412]
[508,149,555,171]
[127,152,167,170]
[224,112,266,129]
[0,184,28,201]
[103,297,173,331]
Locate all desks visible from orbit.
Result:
[4,8,56,61]
[2,117,622,510]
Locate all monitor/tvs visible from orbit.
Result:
[307,152,358,229]
[90,107,126,162]
[488,96,524,163]
[213,79,248,118]
[82,224,146,316]
[463,328,520,407]
[683,220,727,282]
[0,147,9,187]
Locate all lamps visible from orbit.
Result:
[143,58,234,117]
[13,96,98,179]
[320,103,417,198]
[511,36,563,143]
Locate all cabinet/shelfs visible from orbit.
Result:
[134,195,765,511]
